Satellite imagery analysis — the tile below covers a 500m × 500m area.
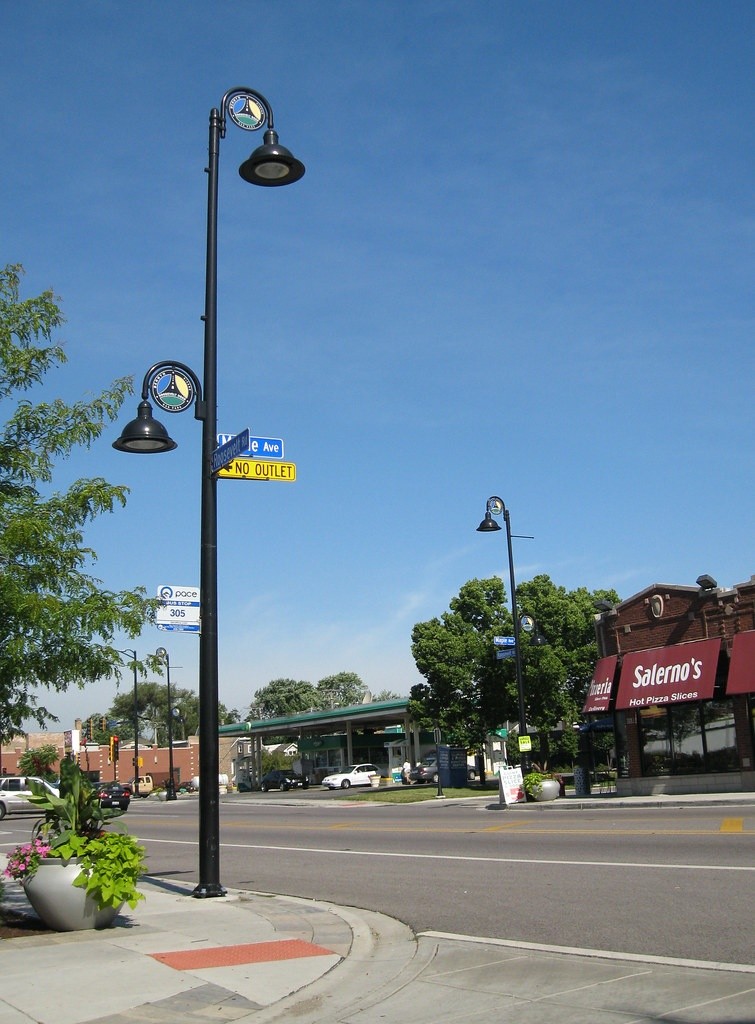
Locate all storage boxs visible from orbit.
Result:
[381,778,393,785]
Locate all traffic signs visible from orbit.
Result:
[210,427,297,481]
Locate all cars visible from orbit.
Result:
[409,758,477,784]
[260,770,309,792]
[321,763,384,790]
[92,781,130,811]
[175,781,198,792]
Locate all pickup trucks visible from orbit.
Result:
[121,775,153,795]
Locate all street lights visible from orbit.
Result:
[151,647,178,800]
[113,84,307,898]
[476,495,533,772]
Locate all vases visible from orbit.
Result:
[24,857,125,932]
[219,786,238,795]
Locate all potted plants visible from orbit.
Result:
[524,759,560,801]
[148,785,168,801]
[368,774,381,787]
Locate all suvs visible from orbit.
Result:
[0,777,60,820]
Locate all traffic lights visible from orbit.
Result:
[76,752,81,766]
[113,736,119,760]
[99,716,104,731]
[87,718,92,741]
[132,757,138,766]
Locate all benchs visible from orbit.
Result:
[593,770,619,796]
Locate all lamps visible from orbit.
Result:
[594,599,612,612]
[696,574,717,590]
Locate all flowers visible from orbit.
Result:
[3,837,50,887]
